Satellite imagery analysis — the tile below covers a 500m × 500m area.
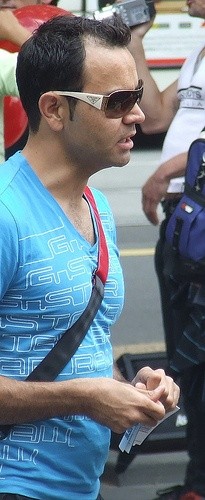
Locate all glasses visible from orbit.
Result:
[47,79,143,118]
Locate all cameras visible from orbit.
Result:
[93,0,156,28]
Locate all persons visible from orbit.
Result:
[0,17,181,500]
[106,0,205,500]
[0,0,57,163]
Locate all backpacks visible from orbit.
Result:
[165,127,205,264]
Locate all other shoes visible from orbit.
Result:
[152,485,205,500]
